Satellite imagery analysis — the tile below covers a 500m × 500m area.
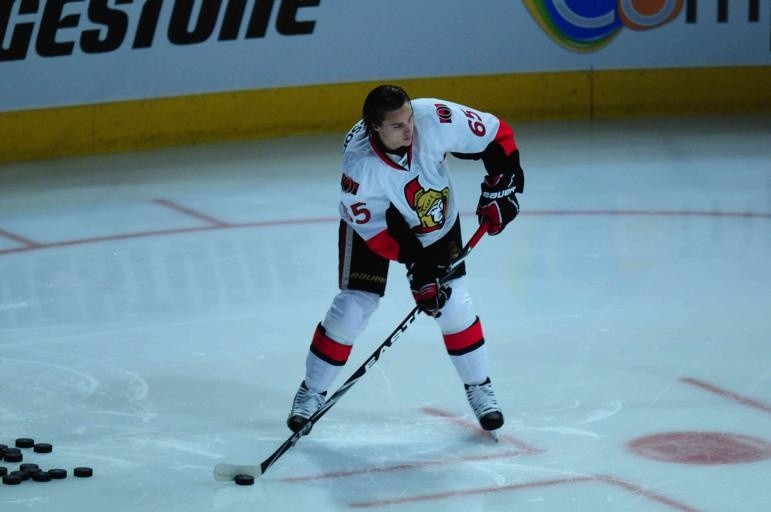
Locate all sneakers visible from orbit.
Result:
[287,380,328,437]
[464,377,506,431]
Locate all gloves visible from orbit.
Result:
[405,260,452,319]
[474,172,522,236]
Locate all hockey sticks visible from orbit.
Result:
[214,221,489,479]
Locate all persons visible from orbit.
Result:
[285,81,526,436]
